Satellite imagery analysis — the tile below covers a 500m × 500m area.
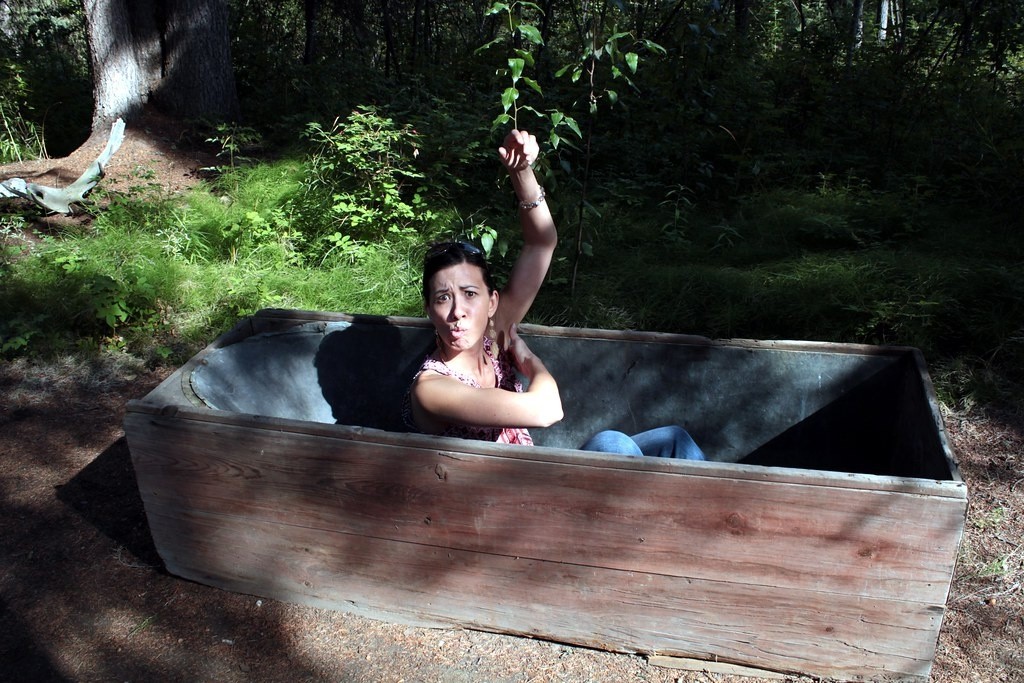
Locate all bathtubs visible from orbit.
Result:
[119,305,972,683]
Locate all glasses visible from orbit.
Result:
[425,241,489,270]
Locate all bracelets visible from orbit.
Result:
[520,185,544,208]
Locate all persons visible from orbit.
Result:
[407,129,706,462]
